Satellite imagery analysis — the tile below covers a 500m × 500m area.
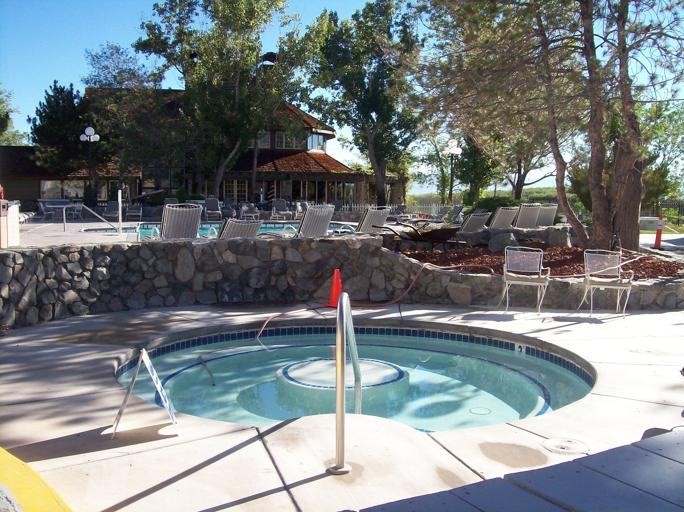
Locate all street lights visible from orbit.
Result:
[443,138,462,203]
[79,126,99,205]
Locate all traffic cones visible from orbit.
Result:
[650,212,666,251]
[321,266,344,310]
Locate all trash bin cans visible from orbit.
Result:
[0,199,21,249]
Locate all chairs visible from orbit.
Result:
[0,429,684,512]
[514,203,541,241]
[576,247,633,314]
[480,206,520,249]
[430,203,490,225]
[296,204,335,238]
[356,206,391,233]
[446,212,492,256]
[160,202,204,240]
[217,217,261,239]
[495,245,551,312]
[32,195,430,226]
[539,203,559,230]
